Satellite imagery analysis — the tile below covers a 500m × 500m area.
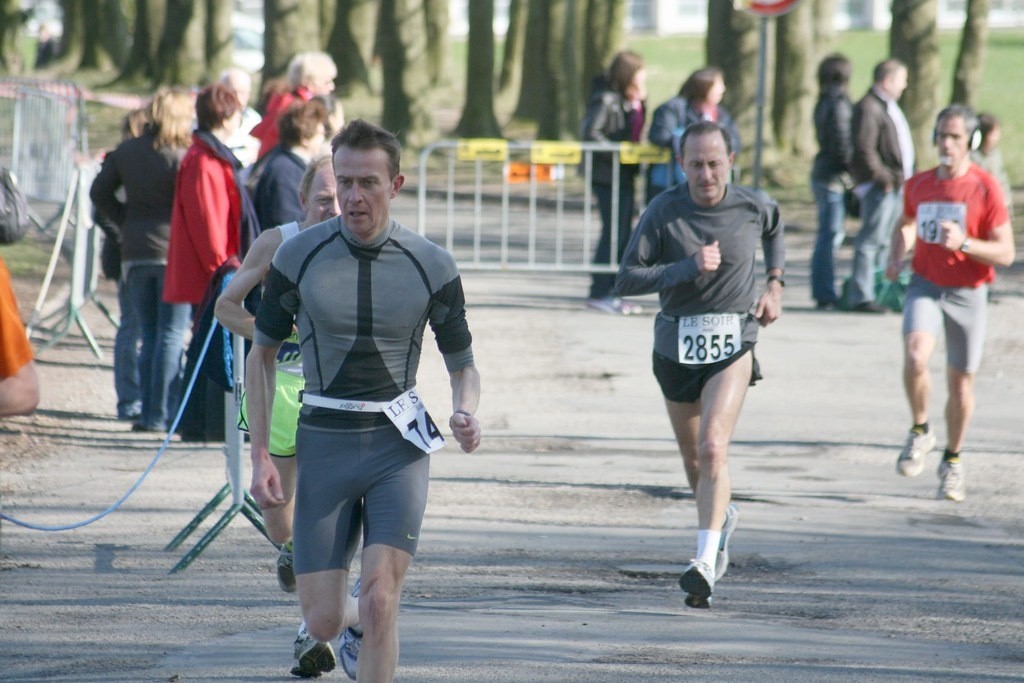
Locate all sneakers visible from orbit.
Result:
[897,428,936,479]
[678,559,713,608]
[288,627,336,678]
[713,504,739,580]
[589,293,642,316]
[938,457,967,502]
[276,540,295,593]
[339,578,361,678]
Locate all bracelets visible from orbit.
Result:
[767,275,785,287]
[960,238,971,253]
[449,410,471,430]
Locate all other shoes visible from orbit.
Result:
[131,423,146,431]
[854,302,885,313]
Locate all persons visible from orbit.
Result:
[89,50,345,443]
[809,51,855,312]
[577,50,648,314]
[969,112,1015,223]
[643,66,741,209]
[838,59,916,314]
[0,252,41,418]
[245,117,480,683]
[615,121,786,607]
[885,105,1016,501]
[214,152,341,679]
